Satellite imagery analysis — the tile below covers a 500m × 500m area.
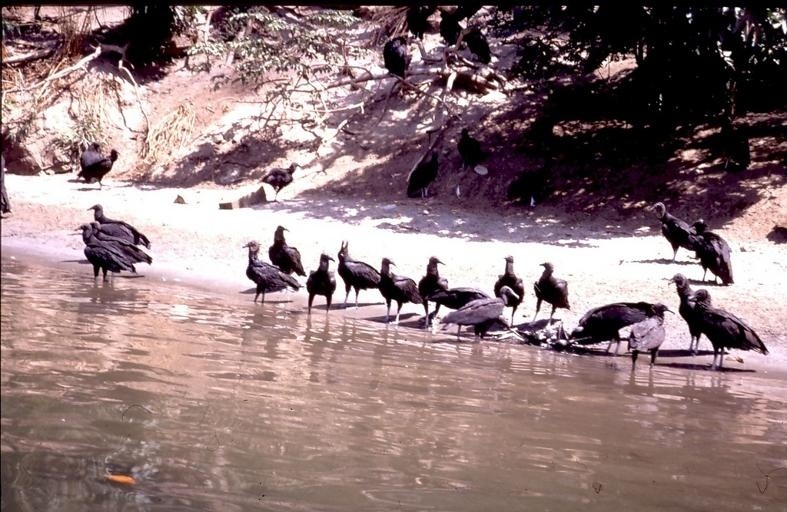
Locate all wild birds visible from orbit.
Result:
[567,301,676,369]
[667,273,770,372]
[241,225,309,304]
[501,166,552,209]
[306,241,378,315]
[76,142,121,190]
[382,5,491,79]
[256,162,302,204]
[407,127,482,198]
[649,201,734,286]
[74,203,154,283]
[379,254,570,342]
